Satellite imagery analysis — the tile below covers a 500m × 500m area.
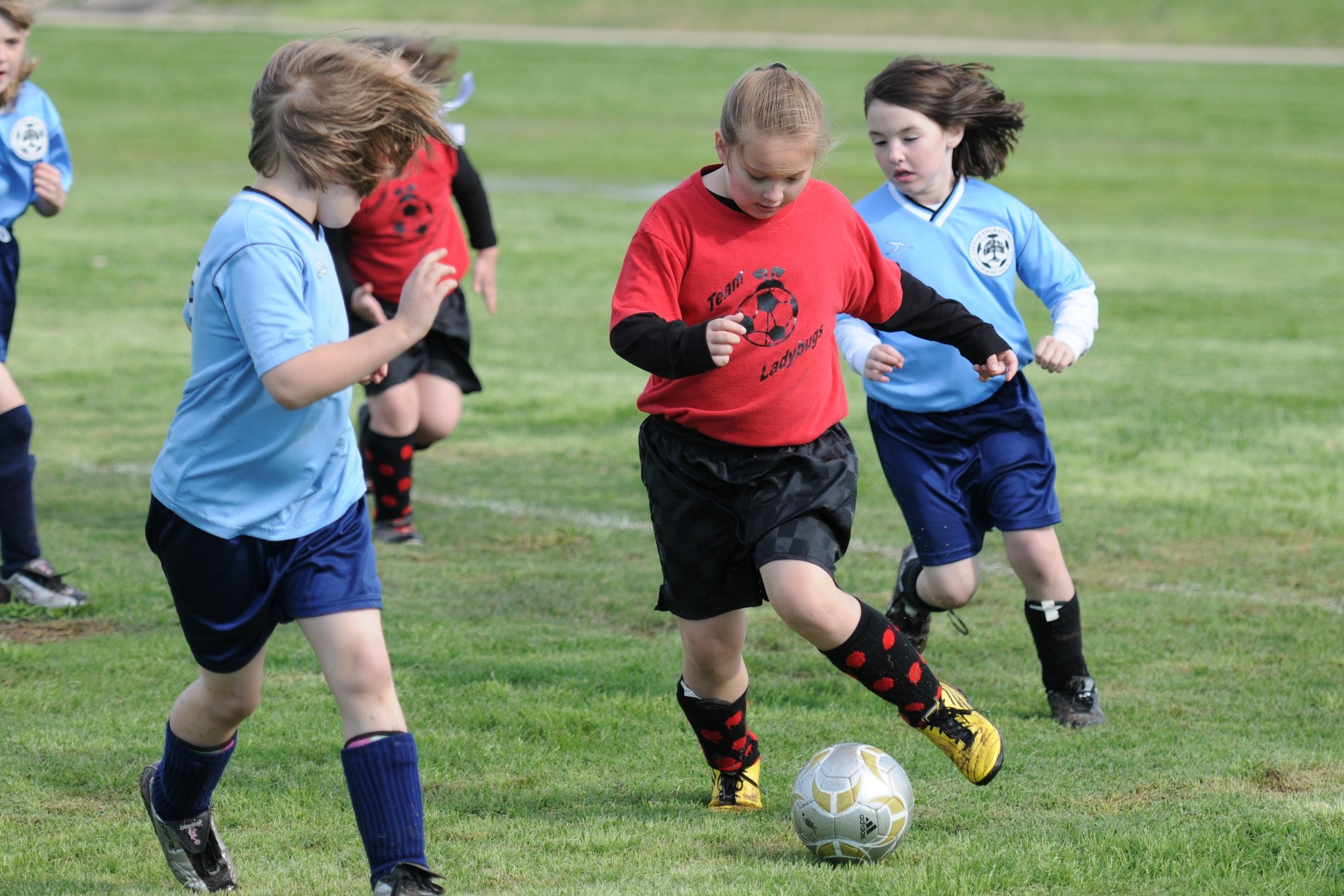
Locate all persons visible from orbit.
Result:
[829,54,1113,727]
[608,59,1005,814]
[136,37,461,896]
[0,1,93,614]
[314,37,506,561]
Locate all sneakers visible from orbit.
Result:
[885,542,931,655]
[1044,676,1107,731]
[357,402,382,493]
[913,680,1004,787]
[373,862,447,896]
[374,513,422,548]
[138,757,237,894]
[0,555,88,609]
[707,757,763,814]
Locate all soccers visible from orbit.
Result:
[789,741,918,865]
